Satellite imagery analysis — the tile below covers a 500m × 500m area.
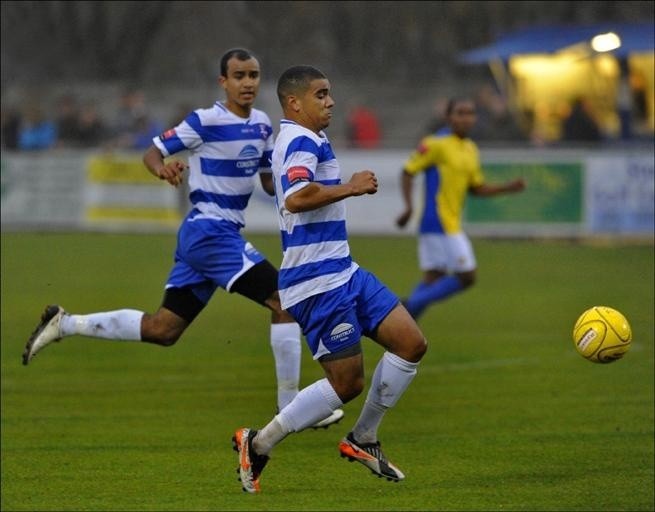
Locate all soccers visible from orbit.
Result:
[572,306,631,363]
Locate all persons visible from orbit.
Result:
[232,66,427,495]
[0,0,653,150]
[23,48,344,430]
[396,100,526,317]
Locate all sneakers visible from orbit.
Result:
[337,432,405,482]
[308,408,344,430]
[22,305,66,366]
[232,427,270,492]
